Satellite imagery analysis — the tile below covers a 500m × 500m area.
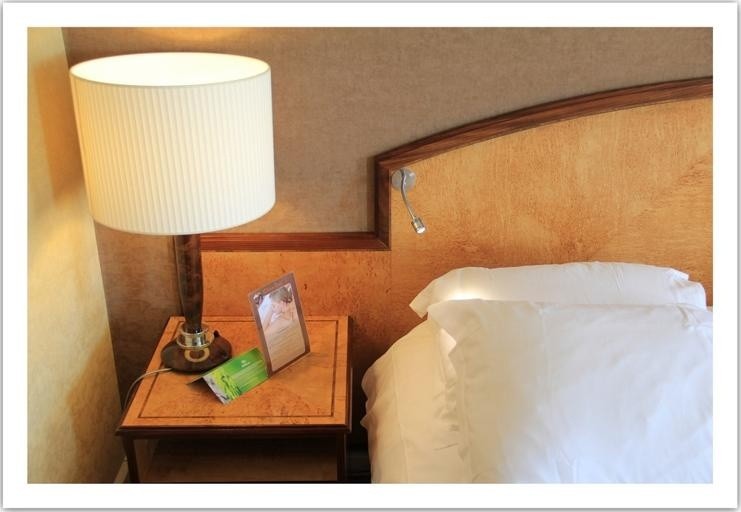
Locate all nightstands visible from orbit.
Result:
[120,316,354,484]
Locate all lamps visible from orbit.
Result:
[69,53,276,371]
[391,169,426,233]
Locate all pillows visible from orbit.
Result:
[430,299,713,484]
[410,264,706,414]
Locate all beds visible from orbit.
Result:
[203,74,713,484]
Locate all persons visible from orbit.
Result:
[263,287,297,333]
